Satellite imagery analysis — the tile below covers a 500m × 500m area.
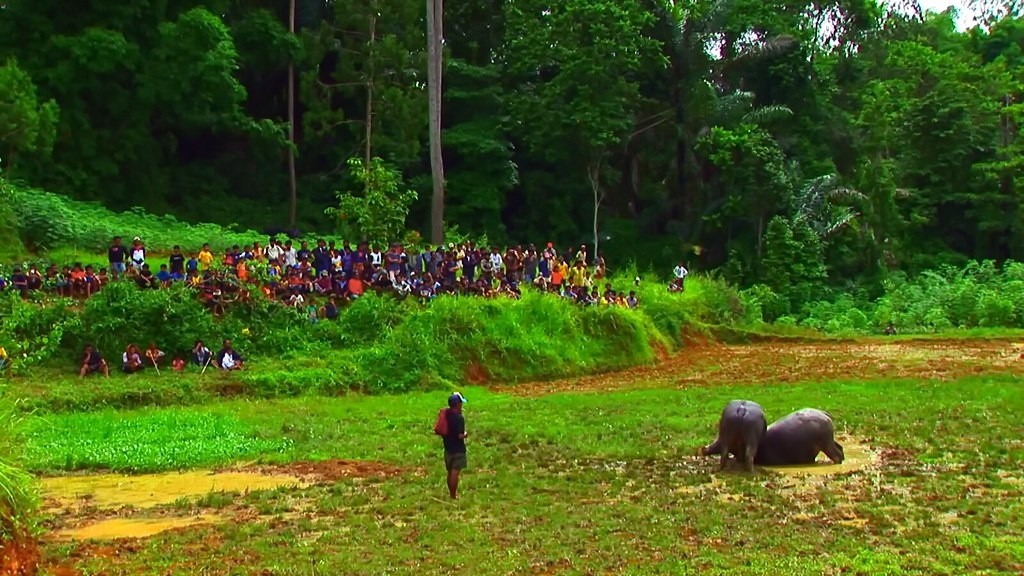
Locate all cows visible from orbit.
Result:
[703,398,845,473]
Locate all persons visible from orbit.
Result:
[667,260,689,293]
[533,242,640,308]
[221,237,538,324]
[438,391,469,500]
[0,337,14,379]
[0,235,226,317]
[79,337,245,378]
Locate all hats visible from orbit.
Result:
[133,237,141,241]
[448,243,455,248]
[321,269,328,276]
[410,271,416,276]
[581,245,586,249]
[547,243,552,248]
[448,392,467,405]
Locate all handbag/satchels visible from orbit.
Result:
[434,408,449,436]
